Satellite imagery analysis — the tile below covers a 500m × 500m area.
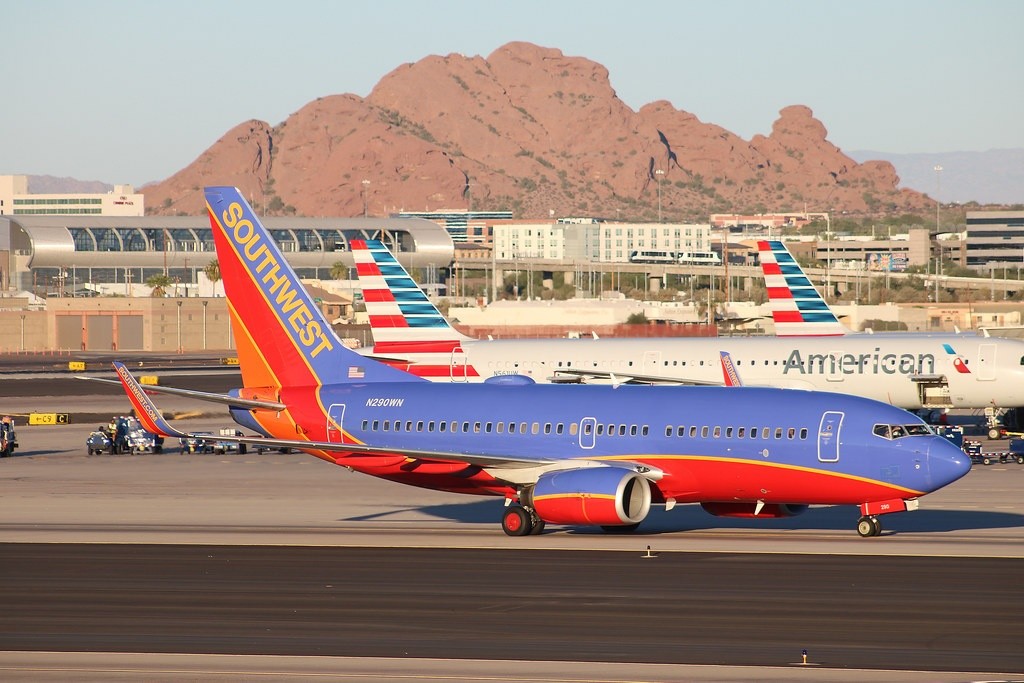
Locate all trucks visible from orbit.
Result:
[0,416,19,455]
[930,422,1024,466]
[185,427,248,456]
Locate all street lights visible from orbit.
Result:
[20,315,25,351]
[201,300,208,350]
[653,169,665,222]
[934,164,943,273]
[177,300,183,353]
[820,214,832,301]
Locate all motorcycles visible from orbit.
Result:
[86,431,115,455]
[108,415,139,435]
[126,419,164,454]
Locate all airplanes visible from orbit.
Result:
[109,181,974,537]
[326,229,1024,428]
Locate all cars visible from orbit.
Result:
[70,287,101,298]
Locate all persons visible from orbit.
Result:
[910,428,917,432]
[884,426,901,436]
[107,419,118,441]
